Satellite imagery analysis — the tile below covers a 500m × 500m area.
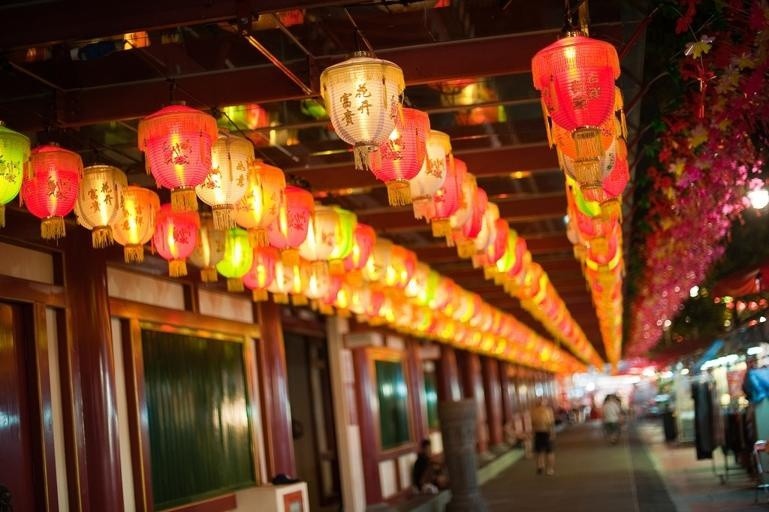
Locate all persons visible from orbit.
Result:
[560,393,627,444]
[528,394,556,477]
[407,437,448,498]
[501,416,534,459]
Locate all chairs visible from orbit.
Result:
[751,438,769,505]
